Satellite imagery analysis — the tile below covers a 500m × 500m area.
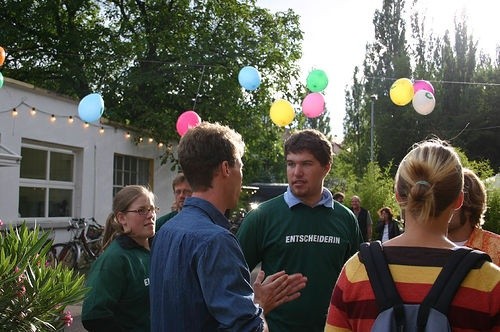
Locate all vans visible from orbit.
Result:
[243,183,290,217]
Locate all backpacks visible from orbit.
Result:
[359,239,492,332]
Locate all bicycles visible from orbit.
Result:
[45,216,106,280]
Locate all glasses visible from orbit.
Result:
[120,205,159,216]
[173,189,192,196]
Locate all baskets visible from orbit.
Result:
[86,224,103,239]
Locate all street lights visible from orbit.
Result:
[369,93,378,164]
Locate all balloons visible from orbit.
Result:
[306,69,329,93]
[238,66,261,91]
[390,78,436,115]
[176,111,201,136]
[269,99,295,127]
[302,92,325,118]
[0,47,5,88]
[78,93,104,122]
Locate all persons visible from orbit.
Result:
[332,192,401,244]
[148,173,194,250]
[81,185,160,332]
[149,121,308,332]
[236,129,364,332]
[324,134,500,332]
[171,201,177,212]
[446,168,500,268]
